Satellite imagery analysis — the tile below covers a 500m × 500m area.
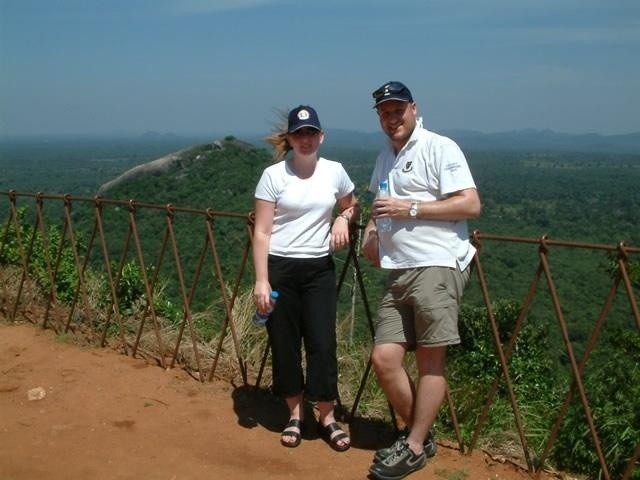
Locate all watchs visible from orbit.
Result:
[409,200,418,220]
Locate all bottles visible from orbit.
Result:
[375,179,393,233]
[250,291,280,327]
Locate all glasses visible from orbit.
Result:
[373,86,406,98]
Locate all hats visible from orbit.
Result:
[288,105,321,134]
[372,81,412,109]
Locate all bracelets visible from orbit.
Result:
[337,212,350,222]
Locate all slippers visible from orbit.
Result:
[317,422,351,451]
[280,420,303,447]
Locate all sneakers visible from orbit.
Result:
[368,425,436,479]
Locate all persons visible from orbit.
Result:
[361,80,482,480]
[252,105,360,451]
